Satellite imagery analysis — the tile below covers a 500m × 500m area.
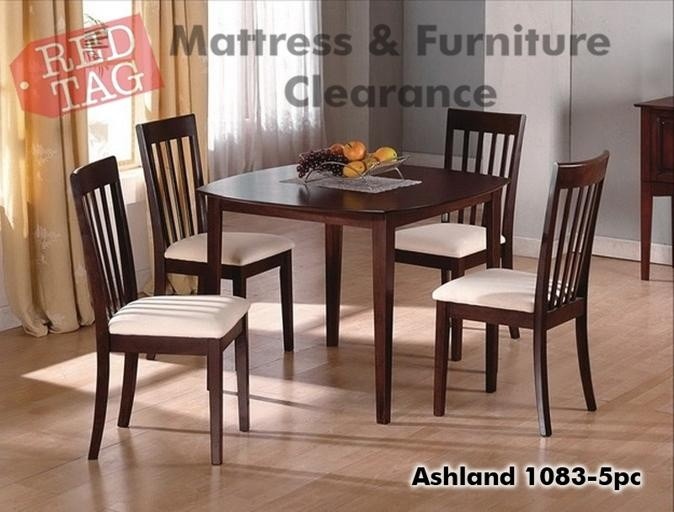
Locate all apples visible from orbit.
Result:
[329,140,397,177]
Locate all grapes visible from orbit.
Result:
[297,149,347,177]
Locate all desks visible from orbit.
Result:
[194,161,514,424]
[632,95,674,281]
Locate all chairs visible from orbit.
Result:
[135,113,300,392]
[430,150,610,437]
[394,107,527,362]
[69,155,253,466]
